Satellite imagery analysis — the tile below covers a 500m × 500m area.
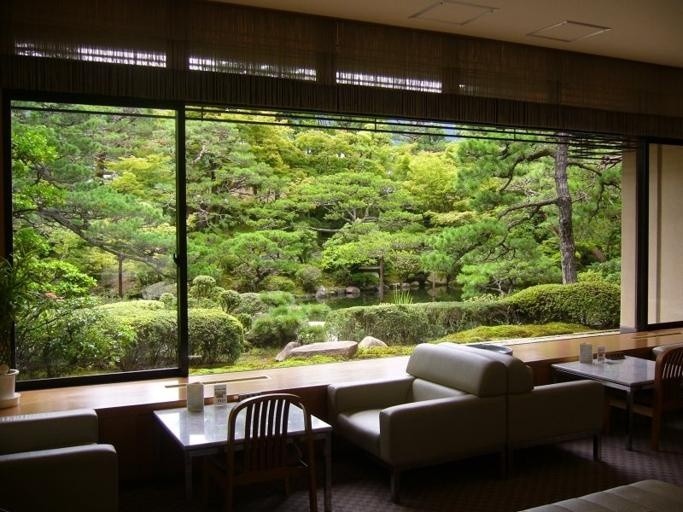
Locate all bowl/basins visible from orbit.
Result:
[465,340,513,358]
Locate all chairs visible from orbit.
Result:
[0,409,118,512]
[610,344,683,458]
[227,395,318,512]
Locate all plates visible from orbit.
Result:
[604,355,628,365]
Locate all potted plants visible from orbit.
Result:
[0,247,39,410]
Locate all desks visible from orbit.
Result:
[154,401,334,512]
[549,354,681,452]
